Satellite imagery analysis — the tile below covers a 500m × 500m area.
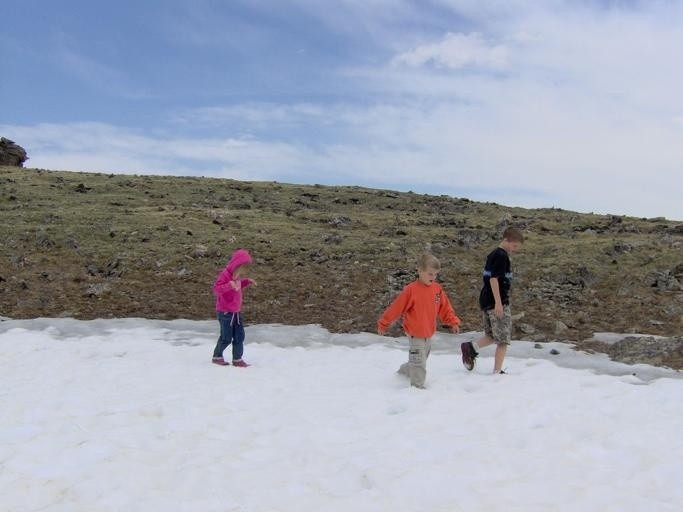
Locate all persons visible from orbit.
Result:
[209,249,259,368]
[376,255,458,388]
[462,226,524,375]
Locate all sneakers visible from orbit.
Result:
[211,357,229,367]
[461,341,478,372]
[233,360,251,368]
